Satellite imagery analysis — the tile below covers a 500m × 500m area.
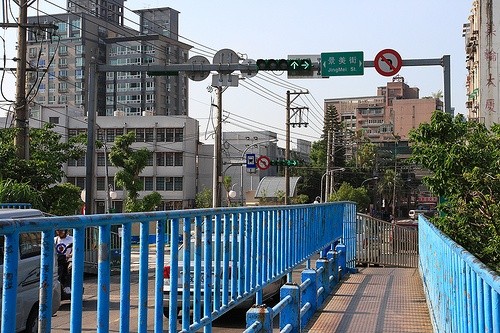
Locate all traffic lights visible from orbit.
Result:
[256,58,312,71]
[270,159,297,166]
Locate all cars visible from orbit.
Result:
[157,218,289,320]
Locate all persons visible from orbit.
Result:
[54,230,73,294]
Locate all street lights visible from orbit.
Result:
[241,139,279,206]
[360,177,379,187]
[321,168,345,203]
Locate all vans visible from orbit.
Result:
[0,209,61,333]
[356,213,382,266]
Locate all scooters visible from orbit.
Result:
[55,243,84,300]
